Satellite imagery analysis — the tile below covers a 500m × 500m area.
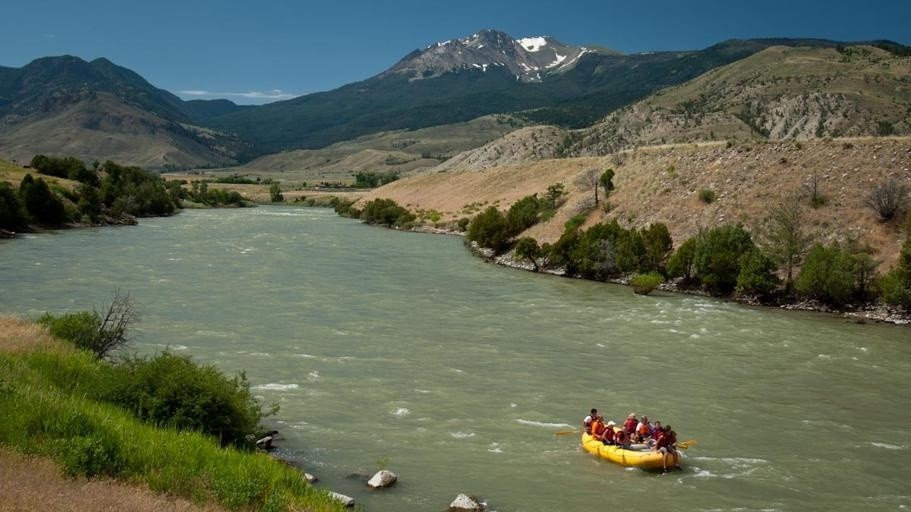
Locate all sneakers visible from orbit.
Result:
[663,463,683,474]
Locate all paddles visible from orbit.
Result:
[676,443,688,449]
[680,441,696,445]
[556,430,590,434]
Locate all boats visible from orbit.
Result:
[582,425,681,468]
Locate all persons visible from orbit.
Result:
[634,415,654,444]
[614,424,636,448]
[646,420,682,474]
[622,412,637,441]
[602,420,617,445]
[592,415,606,440]
[583,408,598,435]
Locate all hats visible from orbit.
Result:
[607,420,617,425]
